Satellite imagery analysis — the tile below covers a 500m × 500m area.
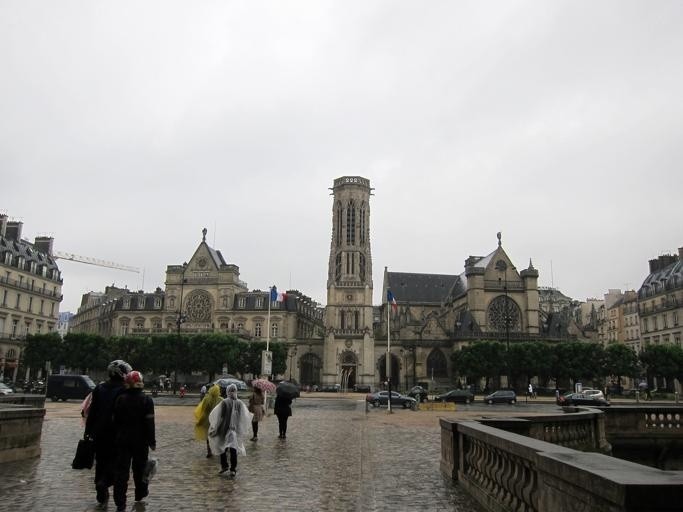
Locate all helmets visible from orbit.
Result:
[108,359,133,382]
[124,370,145,388]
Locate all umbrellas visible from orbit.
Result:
[638,383,648,386]
[275,380,302,398]
[251,379,277,393]
[214,379,249,390]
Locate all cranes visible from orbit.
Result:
[51,248,141,278]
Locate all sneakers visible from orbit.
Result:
[218,467,236,476]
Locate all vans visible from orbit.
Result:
[46,374,95,401]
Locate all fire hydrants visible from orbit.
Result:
[151,384,157,397]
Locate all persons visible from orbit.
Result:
[79,380,108,420]
[84,358,132,511]
[533,384,537,399]
[157,373,207,400]
[247,388,266,441]
[641,387,653,401]
[273,393,293,438]
[194,387,225,459]
[213,385,250,476]
[528,383,533,399]
[115,369,156,510]
[603,385,609,400]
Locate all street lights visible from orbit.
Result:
[494,265,516,349]
[171,268,193,393]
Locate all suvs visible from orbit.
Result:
[580,390,603,397]
[484,390,516,404]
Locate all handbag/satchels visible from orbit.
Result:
[72,437,95,469]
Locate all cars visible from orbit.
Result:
[366,390,416,408]
[407,386,430,403]
[1,382,12,396]
[204,378,249,396]
[557,393,610,406]
[646,387,673,399]
[434,389,474,403]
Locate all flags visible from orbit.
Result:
[270,289,287,302]
[387,289,398,318]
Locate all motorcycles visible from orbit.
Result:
[20,376,45,395]
